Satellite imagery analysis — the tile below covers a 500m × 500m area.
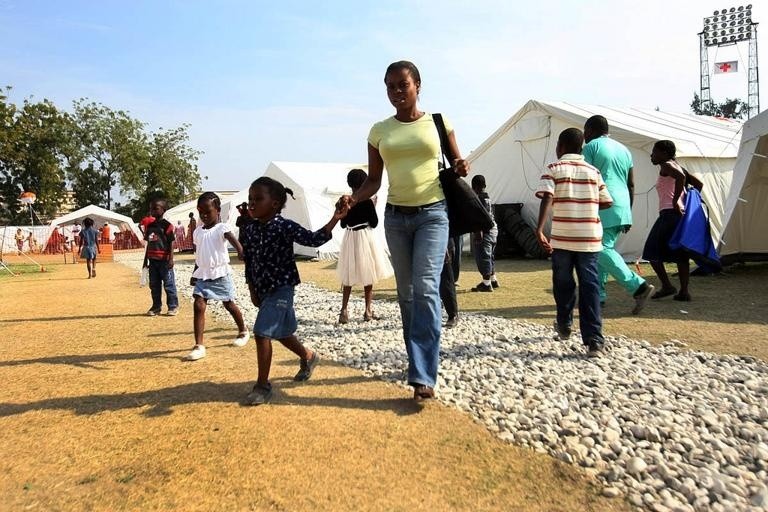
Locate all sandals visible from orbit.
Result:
[413,384,435,401]
[296,351,319,381]
[338,314,348,323]
[364,313,384,321]
[246,381,274,404]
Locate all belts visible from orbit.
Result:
[386,203,438,215]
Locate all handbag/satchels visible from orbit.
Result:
[430,111,495,238]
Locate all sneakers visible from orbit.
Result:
[233,328,251,347]
[147,307,162,316]
[188,345,206,361]
[588,342,607,356]
[167,306,179,316]
[553,320,569,340]
[632,284,654,315]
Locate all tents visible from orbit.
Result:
[54,204,145,264]
[162,192,225,238]
[691,108,768,276]
[260,160,392,262]
[455,99,742,263]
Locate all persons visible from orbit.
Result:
[78,218,101,279]
[236,202,249,261]
[335,61,470,404]
[642,140,703,301]
[580,114,655,314]
[101,224,110,243]
[15,229,24,255]
[174,220,185,253]
[186,192,251,361]
[471,175,500,293]
[534,127,613,358]
[336,169,395,323]
[189,212,196,254]
[138,208,156,236]
[72,220,82,245]
[447,235,464,287]
[439,248,458,327]
[143,197,179,315]
[24,232,34,253]
[240,176,350,405]
